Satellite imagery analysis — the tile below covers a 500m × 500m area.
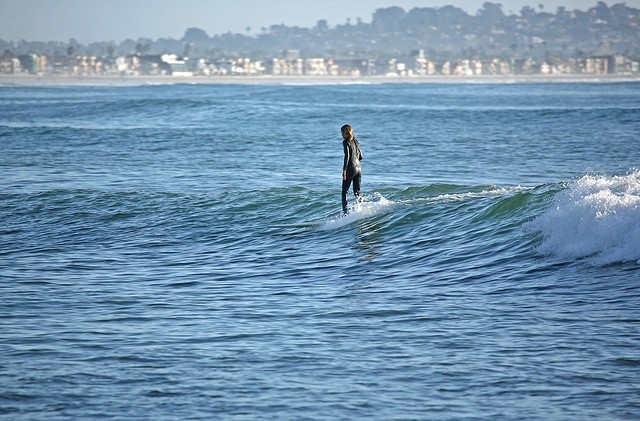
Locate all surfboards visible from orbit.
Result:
[268,221,327,228]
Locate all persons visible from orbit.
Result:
[340,125,363,218]
